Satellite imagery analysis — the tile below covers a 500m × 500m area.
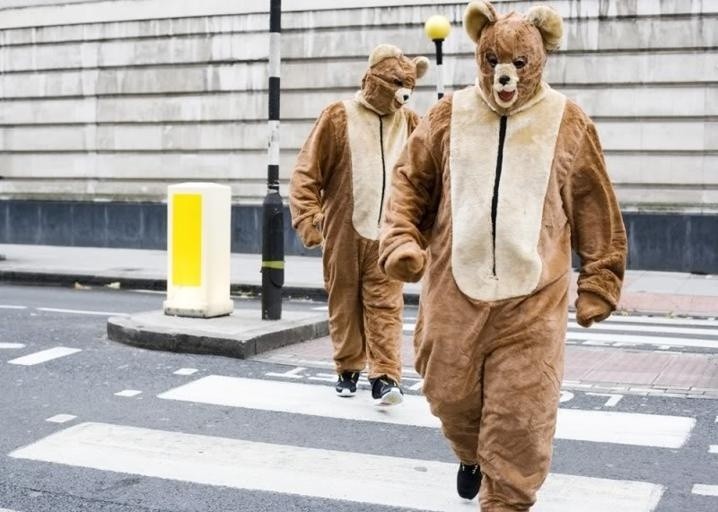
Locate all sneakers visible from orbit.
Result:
[371,375,404,406]
[456,461,483,502]
[334,372,359,396]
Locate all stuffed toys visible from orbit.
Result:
[288,45,430,403]
[377,2,626,512]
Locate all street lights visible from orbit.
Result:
[424,14,450,100]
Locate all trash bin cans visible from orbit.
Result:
[162,180,234,318]
